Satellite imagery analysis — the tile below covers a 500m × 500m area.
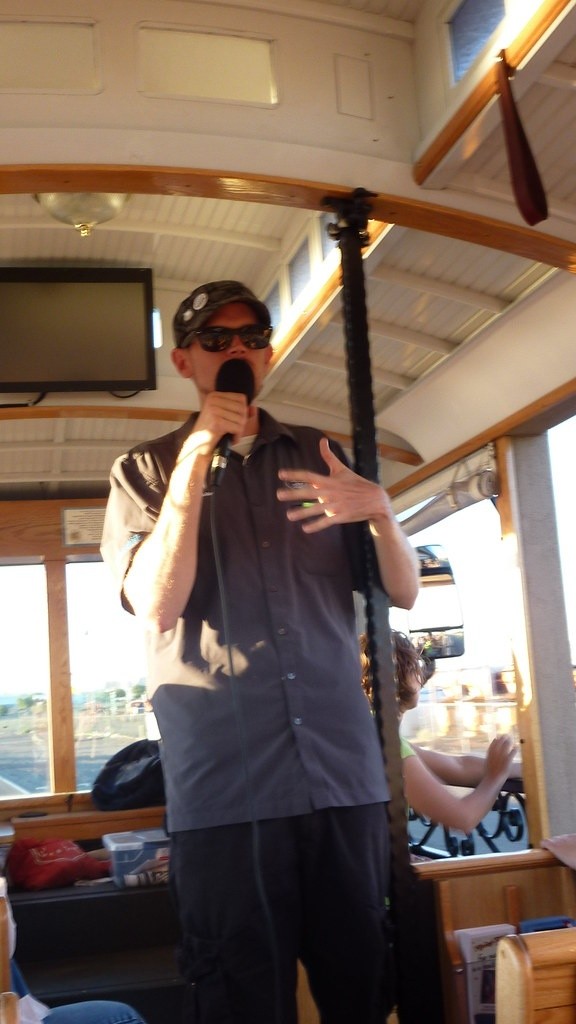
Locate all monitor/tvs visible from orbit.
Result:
[0,266,157,392]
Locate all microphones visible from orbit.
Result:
[210,358,255,486]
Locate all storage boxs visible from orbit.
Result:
[101,824,173,889]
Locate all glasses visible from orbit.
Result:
[180,324,273,352]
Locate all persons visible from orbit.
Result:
[571,665,576,688]
[359,630,518,864]
[98,281,420,1024]
[0,877,146,1024]
[539,833,576,870]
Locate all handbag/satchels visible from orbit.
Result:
[6,836,110,891]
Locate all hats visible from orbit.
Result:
[172,279,271,348]
